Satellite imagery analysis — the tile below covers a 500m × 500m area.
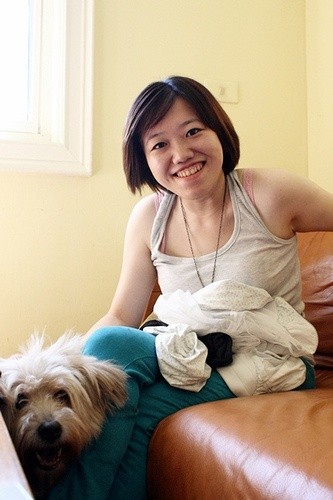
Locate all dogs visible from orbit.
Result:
[0,350,131,484]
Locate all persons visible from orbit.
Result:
[36,74,333,500]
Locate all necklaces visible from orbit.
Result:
[177,174,228,290]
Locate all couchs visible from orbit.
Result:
[142,229,333,500]
